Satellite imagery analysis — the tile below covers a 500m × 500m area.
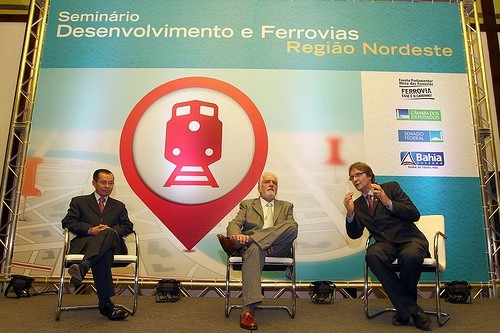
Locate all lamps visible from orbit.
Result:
[156,279,183,301]
[309,281,336,304]
[4,274,35,299]
[443,281,472,304]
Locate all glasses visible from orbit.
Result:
[348,172,366,181]
[98,180,115,186]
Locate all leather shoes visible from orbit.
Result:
[240,309,257,329]
[412,306,431,328]
[392,310,410,326]
[217,233,237,256]
[67,264,86,285]
[98,301,129,321]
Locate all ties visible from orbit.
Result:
[98,197,105,213]
[265,203,274,227]
[366,194,373,211]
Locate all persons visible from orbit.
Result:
[344,162,432,328]
[217,173,298,330]
[62,169,133,321]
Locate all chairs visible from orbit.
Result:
[56,227,140,320]
[225,221,296,320]
[363,214,449,326]
